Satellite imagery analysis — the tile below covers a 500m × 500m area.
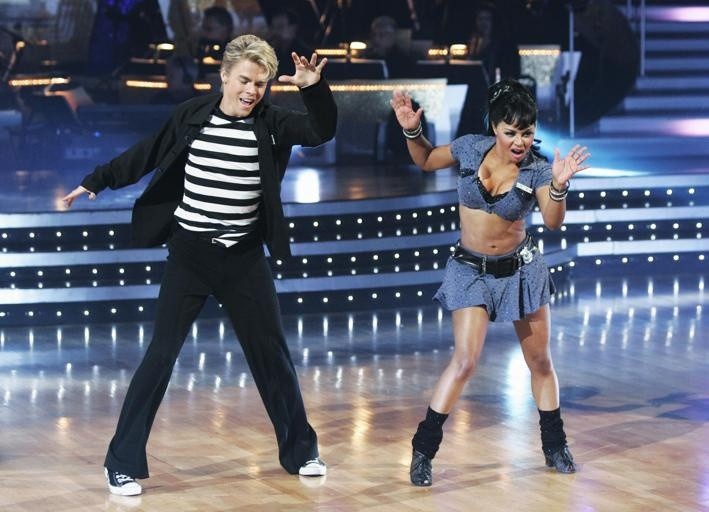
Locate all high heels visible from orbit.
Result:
[543,444,579,474]
[409,445,434,485]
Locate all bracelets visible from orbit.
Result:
[402,122,423,139]
[549,181,570,202]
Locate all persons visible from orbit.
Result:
[61,33,336,495]
[389,79,593,486]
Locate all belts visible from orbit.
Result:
[449,231,537,279]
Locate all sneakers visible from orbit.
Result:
[296,457,329,477]
[102,464,142,497]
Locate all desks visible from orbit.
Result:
[117,76,468,167]
